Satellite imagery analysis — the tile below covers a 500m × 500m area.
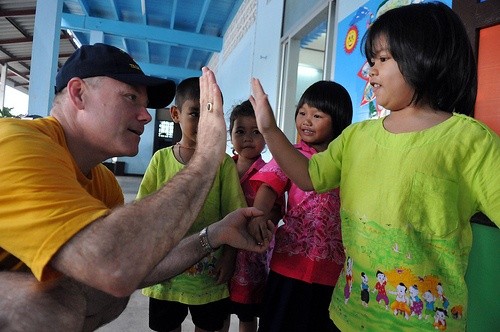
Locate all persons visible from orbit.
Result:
[250,80,353,332]
[0,42,275,332]
[135,77,247,332]
[227,100,285,332]
[250,1,500,332]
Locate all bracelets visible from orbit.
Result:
[199,227,221,253]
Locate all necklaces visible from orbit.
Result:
[177,142,195,150]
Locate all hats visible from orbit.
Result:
[54,43,177,109]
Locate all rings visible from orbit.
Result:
[207,102,213,111]
